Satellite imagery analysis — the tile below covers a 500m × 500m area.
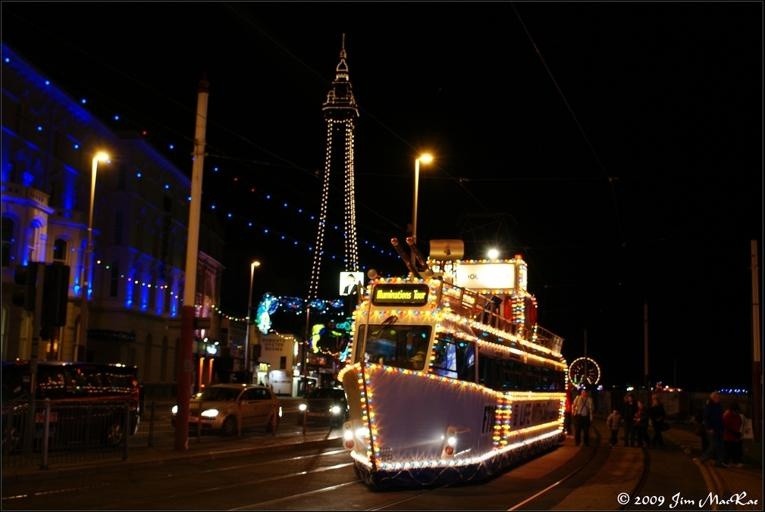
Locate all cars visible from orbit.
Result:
[171,382,281,437]
[297,386,349,429]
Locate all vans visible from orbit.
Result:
[1,359,142,452]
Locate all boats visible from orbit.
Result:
[333,233,578,488]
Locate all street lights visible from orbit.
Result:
[71,149,115,362]
[241,259,263,373]
[409,148,437,268]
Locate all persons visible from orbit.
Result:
[695,392,744,469]
[572,389,593,447]
[344,274,357,295]
[606,392,666,448]
